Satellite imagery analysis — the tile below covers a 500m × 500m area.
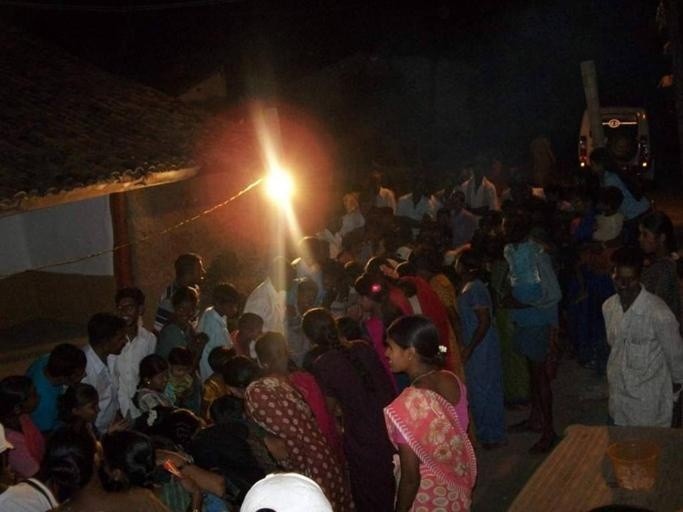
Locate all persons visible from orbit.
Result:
[1,134,683,511]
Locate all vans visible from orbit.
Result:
[579,109,654,178]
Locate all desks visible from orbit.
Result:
[507,422,683,512]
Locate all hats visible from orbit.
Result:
[239,472,333,512]
